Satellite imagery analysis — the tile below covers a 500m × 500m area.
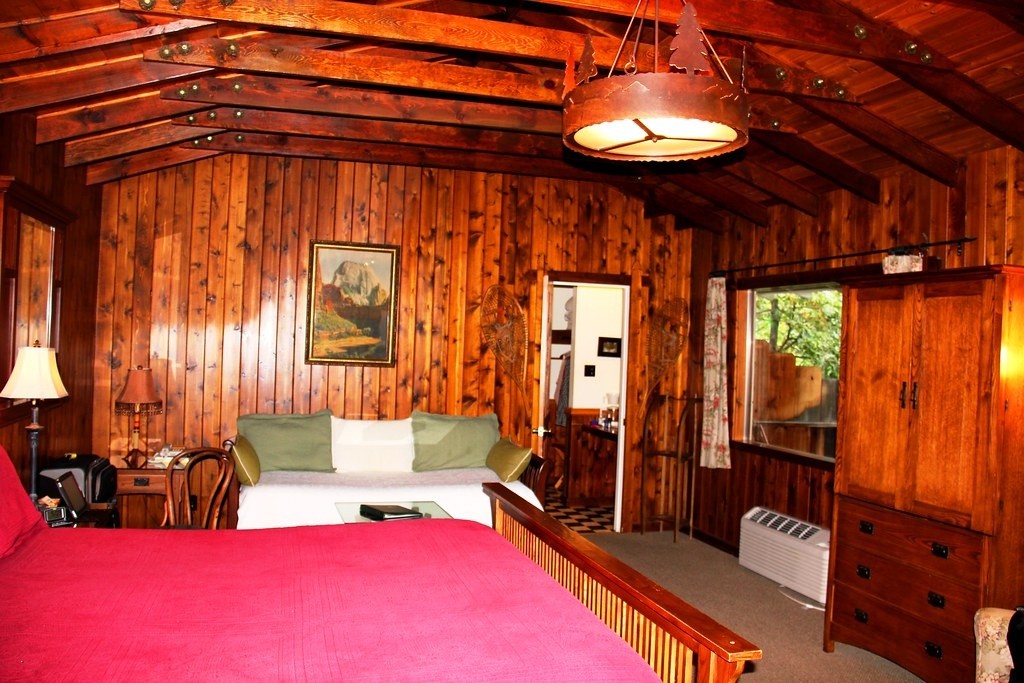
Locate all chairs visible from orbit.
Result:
[160,446,240,530]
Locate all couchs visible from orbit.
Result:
[223,415,555,530]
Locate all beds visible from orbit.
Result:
[0,447,763,683]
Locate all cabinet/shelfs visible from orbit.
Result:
[822,263,1024,683]
[582,423,618,508]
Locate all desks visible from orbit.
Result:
[115,469,187,529]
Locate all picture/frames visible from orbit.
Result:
[304,239,400,368]
[335,501,454,524]
[597,336,622,358]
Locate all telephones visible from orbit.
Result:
[160,444,183,458]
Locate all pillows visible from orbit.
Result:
[412,411,500,472]
[486,435,532,483]
[237,408,337,473]
[231,435,260,487]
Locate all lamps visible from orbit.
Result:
[561,0,749,162]
[0,340,69,493]
[115,365,163,458]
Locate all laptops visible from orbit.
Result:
[55,472,114,523]
